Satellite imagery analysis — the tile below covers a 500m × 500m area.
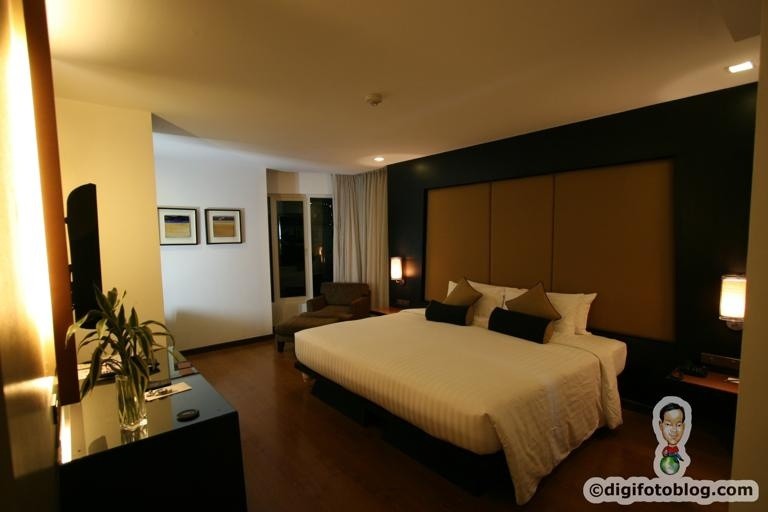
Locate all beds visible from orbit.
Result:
[289,275,671,509]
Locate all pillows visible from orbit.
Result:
[423,279,600,348]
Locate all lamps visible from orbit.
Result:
[387,255,406,287]
[716,271,749,331]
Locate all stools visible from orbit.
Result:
[271,312,344,355]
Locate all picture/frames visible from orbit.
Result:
[204,208,243,245]
[156,206,199,246]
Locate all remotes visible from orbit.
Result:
[96,368,118,377]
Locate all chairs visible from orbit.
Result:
[303,279,371,318]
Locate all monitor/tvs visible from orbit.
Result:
[67,183,103,329]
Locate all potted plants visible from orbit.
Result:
[61,282,182,435]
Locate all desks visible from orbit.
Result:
[53,338,249,511]
[670,360,743,398]
